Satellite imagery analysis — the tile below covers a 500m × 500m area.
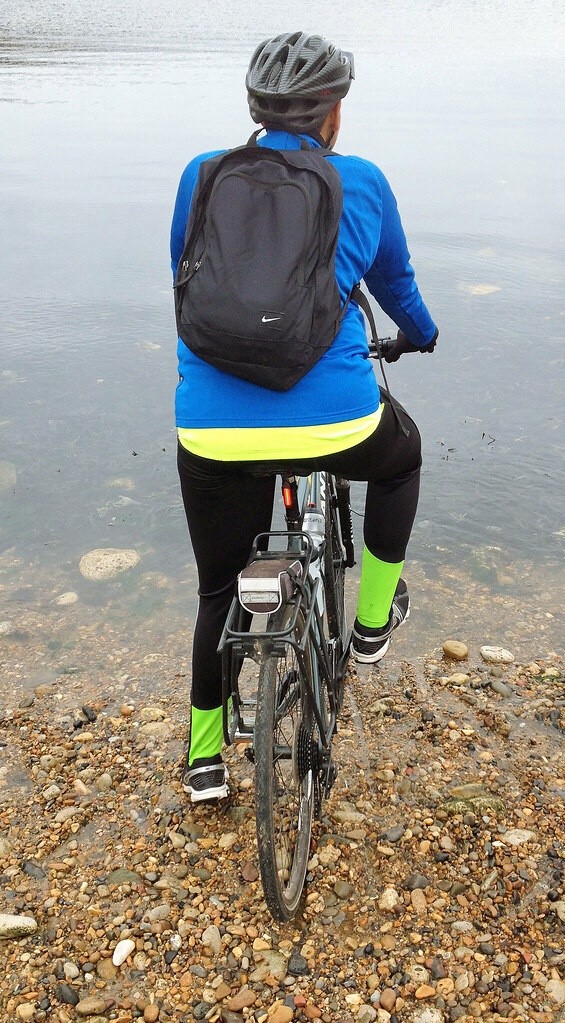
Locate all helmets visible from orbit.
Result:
[246,33,353,133]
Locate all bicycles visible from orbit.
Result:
[176,326,432,928]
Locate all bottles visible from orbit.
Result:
[300,501,325,568]
[299,538,324,617]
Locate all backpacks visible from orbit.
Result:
[172,125,411,437]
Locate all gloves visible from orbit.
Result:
[386,329,438,364]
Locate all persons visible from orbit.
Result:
[171,32,439,801]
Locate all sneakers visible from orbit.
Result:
[181,752,230,803]
[349,578,411,664]
